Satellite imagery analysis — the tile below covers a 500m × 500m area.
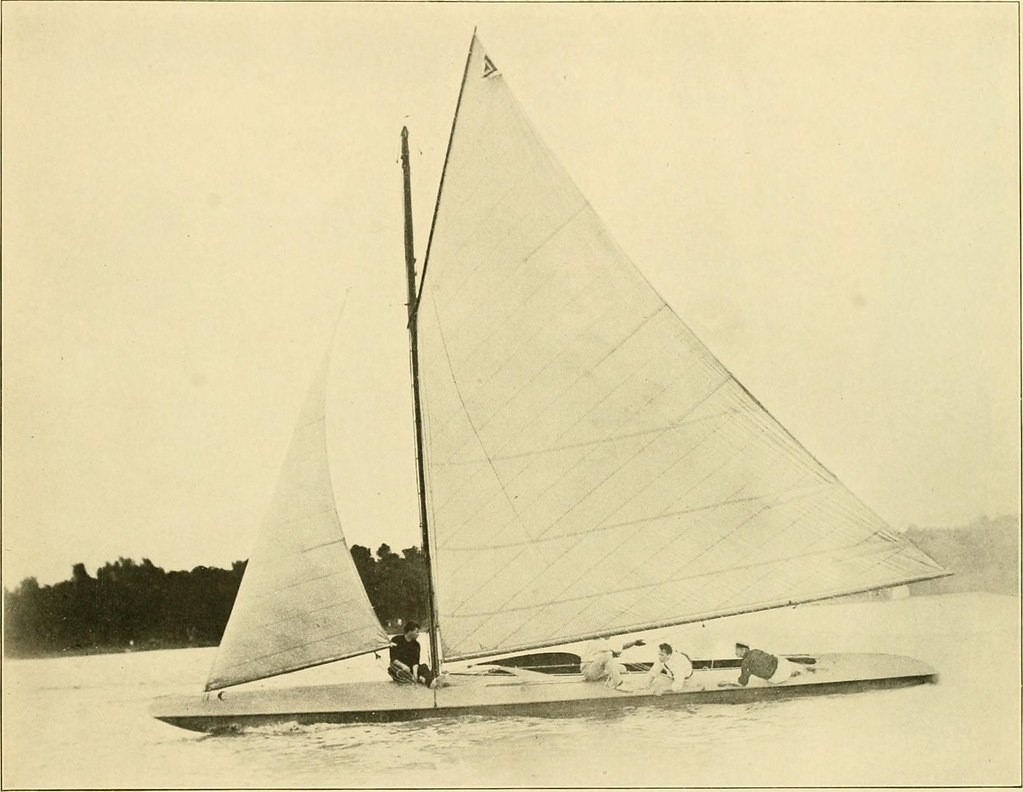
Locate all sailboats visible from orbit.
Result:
[146,24,959,737]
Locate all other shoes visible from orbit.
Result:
[616,684,634,694]
[429,675,444,689]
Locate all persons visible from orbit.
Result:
[389,620,441,687]
[719,641,801,687]
[578,633,647,692]
[643,642,695,692]
